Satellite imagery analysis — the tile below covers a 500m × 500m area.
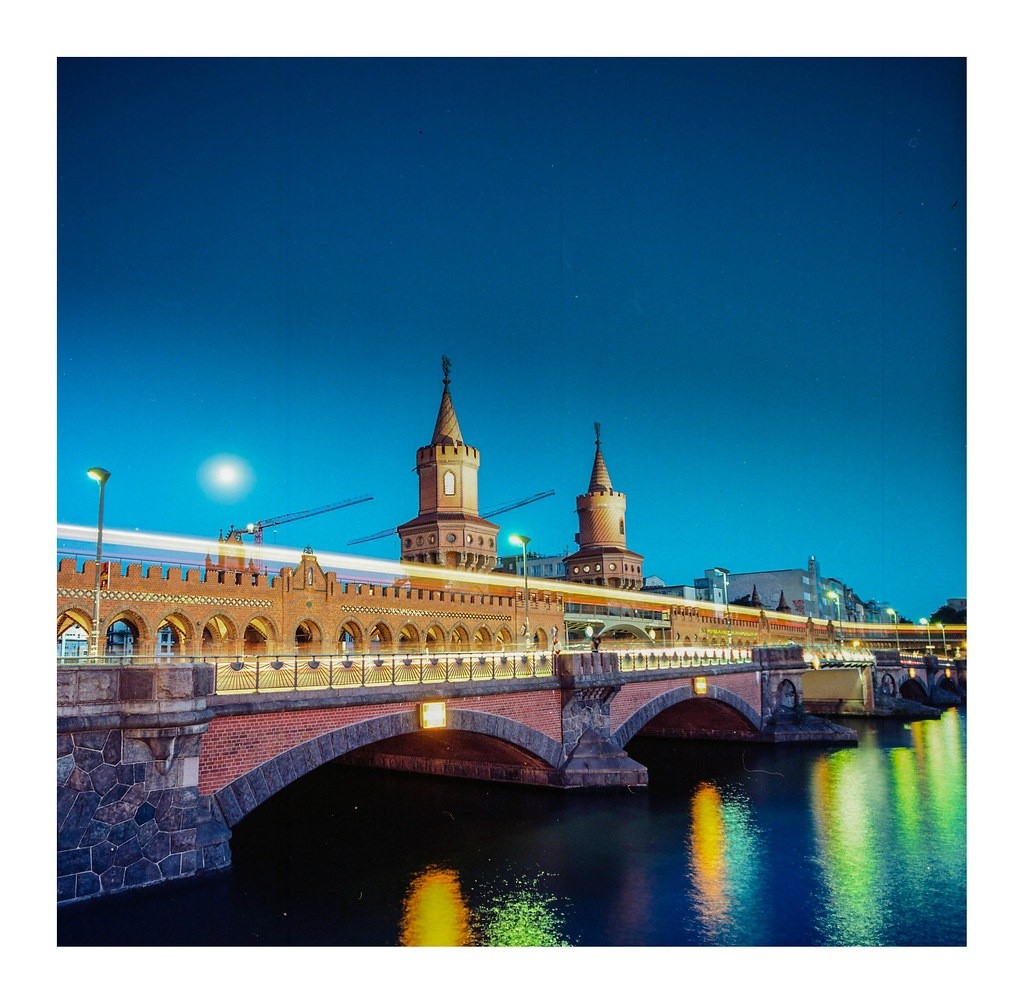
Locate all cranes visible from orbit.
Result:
[350,489,558,548]
[229,492,374,573]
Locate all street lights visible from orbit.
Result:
[936,621,947,659]
[826,591,844,649]
[508,533,532,651]
[886,607,900,650]
[919,618,932,655]
[713,567,734,645]
[84,466,110,658]
